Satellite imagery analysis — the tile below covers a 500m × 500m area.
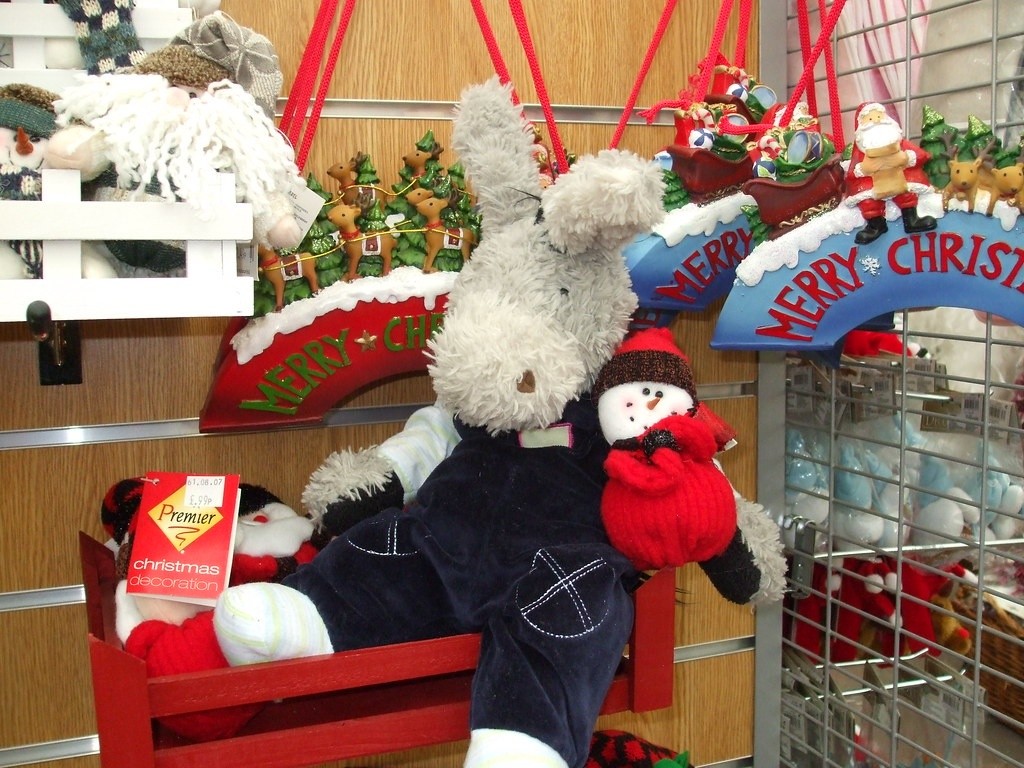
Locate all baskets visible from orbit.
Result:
[953,584,1024,736]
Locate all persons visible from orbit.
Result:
[786,102,821,134]
[846,102,937,245]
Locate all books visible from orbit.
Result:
[125,470,242,607]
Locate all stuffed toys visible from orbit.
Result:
[780,417,1024,664]
[0,0,300,279]
[214,74,786,767]
[101,476,336,742]
[844,312,930,361]
[595,327,735,567]
